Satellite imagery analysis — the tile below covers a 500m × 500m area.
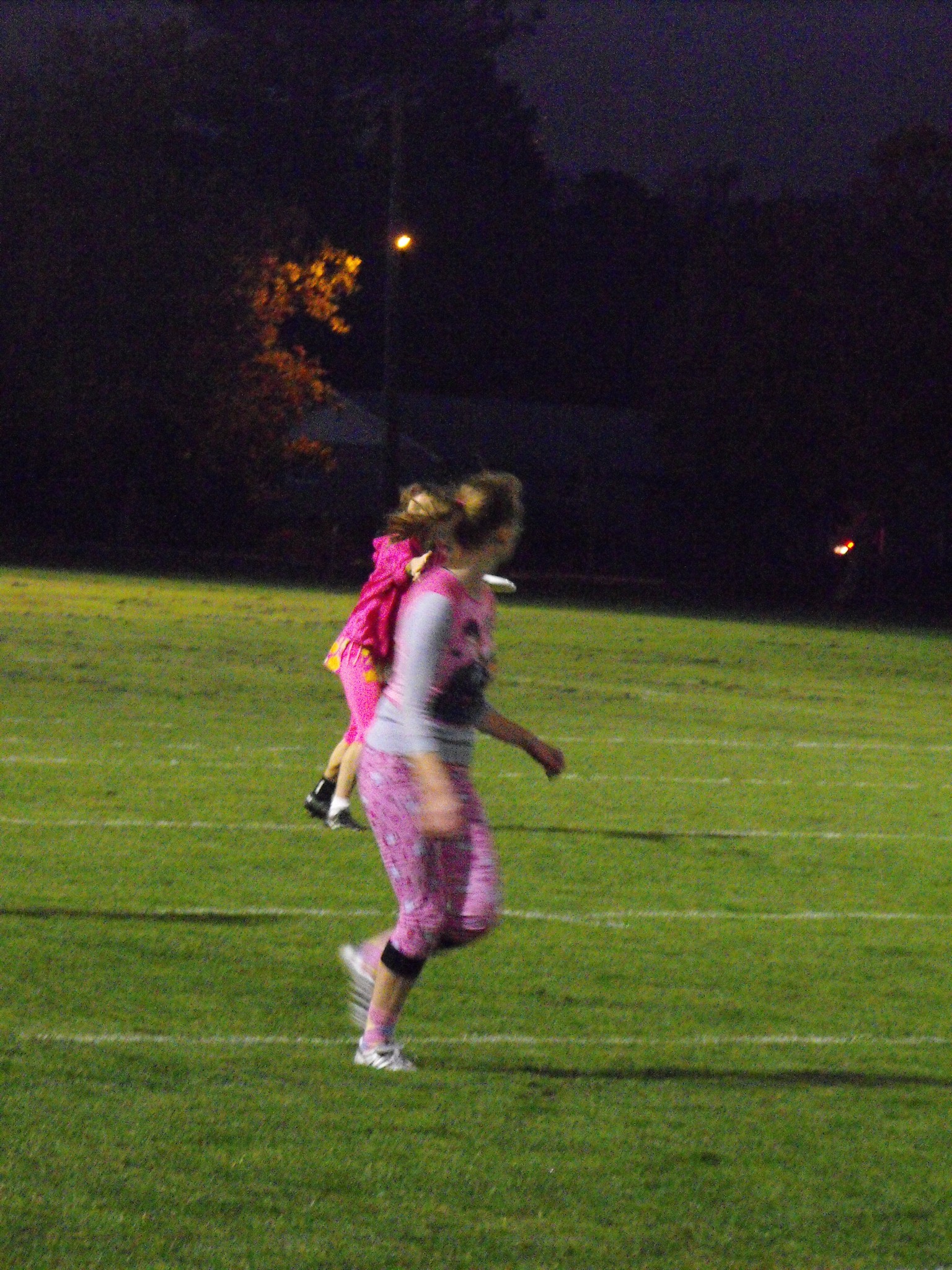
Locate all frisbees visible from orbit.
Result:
[482,573,517,594]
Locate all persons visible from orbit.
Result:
[336,471,566,1073]
[303,484,452,832]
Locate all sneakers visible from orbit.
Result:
[304,795,329,815]
[354,1037,420,1071]
[324,808,366,831]
[340,944,378,1028]
